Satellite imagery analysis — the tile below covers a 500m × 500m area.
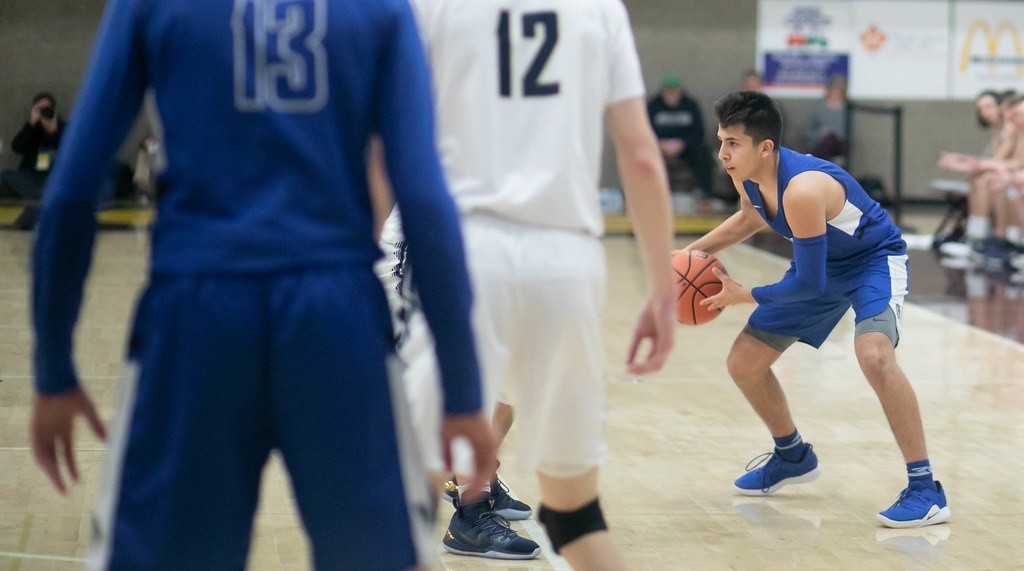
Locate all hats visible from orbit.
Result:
[659,74,680,89]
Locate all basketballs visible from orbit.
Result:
[670,248,731,327]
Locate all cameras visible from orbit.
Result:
[39,106,53,119]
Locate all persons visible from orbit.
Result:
[371,1,679,571]
[27,0,498,571]
[644,76,1024,283]
[12,95,66,230]
[671,89,950,527]
[135,138,161,212]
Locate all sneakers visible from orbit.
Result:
[440,477,533,520]
[442,497,541,559]
[733,442,822,496]
[876,480,951,529]
